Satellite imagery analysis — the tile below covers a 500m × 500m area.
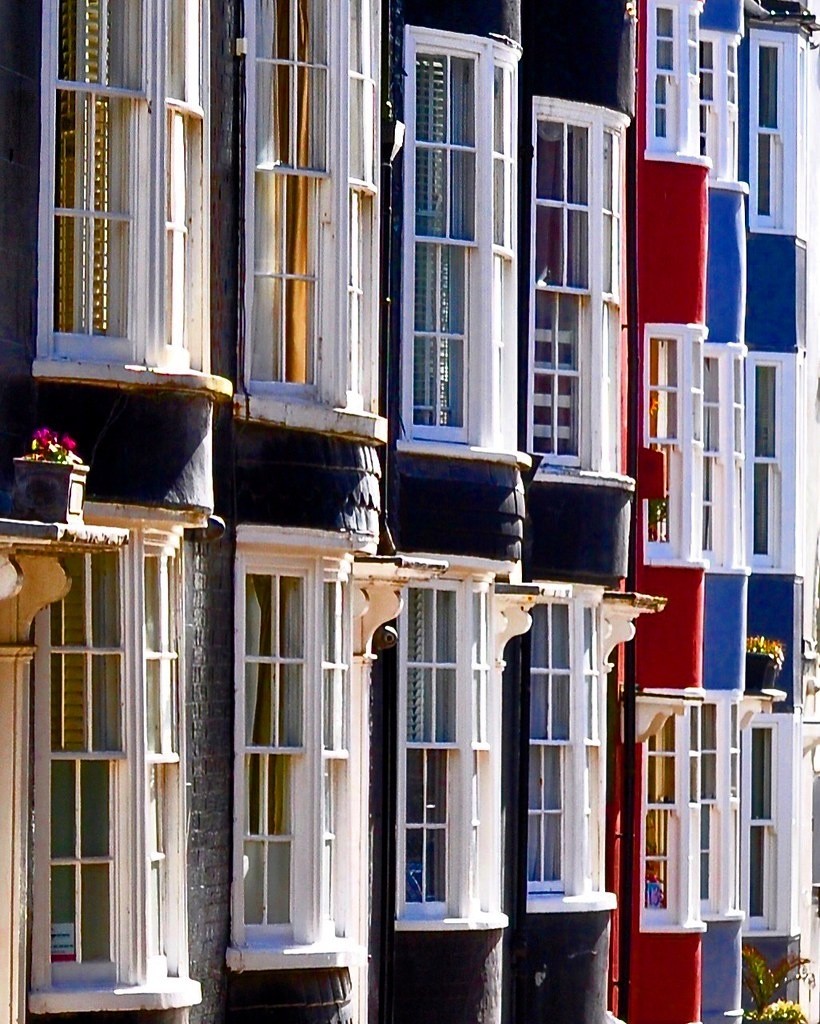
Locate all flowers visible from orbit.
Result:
[12,423,87,464]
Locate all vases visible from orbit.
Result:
[13,459,90,525]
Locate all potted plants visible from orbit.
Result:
[745,635,785,696]
